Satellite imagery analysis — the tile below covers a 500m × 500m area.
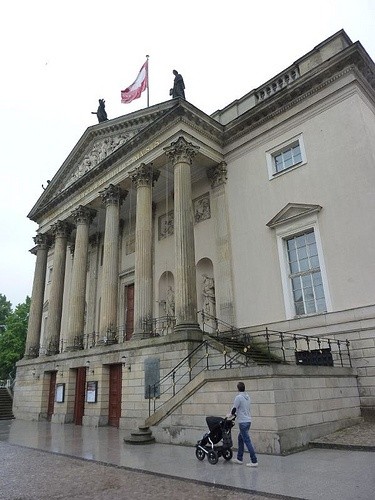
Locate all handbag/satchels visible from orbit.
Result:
[222,421,233,449]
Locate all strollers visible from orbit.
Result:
[194,414,236,465]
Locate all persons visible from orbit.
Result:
[201,271,214,321]
[158,284,176,333]
[226,381,257,467]
[91,99,109,123]
[170,69,186,100]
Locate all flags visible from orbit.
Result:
[120,61,147,105]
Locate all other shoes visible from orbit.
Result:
[246,462,258,467]
[230,459,243,464]
